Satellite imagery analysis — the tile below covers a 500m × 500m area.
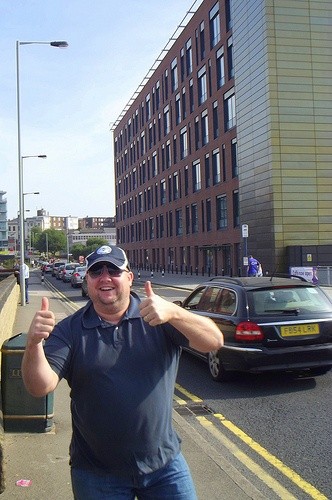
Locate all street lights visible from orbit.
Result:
[18,154,51,305]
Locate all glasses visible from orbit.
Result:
[87,265,121,277]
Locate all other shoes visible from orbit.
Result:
[19,301,29,304]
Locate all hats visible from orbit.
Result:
[85,245,131,275]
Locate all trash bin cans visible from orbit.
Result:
[0,332,53,432]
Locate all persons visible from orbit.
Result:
[18,262,30,305]
[248,255,263,277]
[42,256,60,264]
[22,245,225,500]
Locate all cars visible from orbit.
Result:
[62,263,82,283]
[70,266,87,288]
[35,260,66,277]
[80,270,90,298]
[57,264,65,280]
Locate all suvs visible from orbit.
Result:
[171,273,332,383]
